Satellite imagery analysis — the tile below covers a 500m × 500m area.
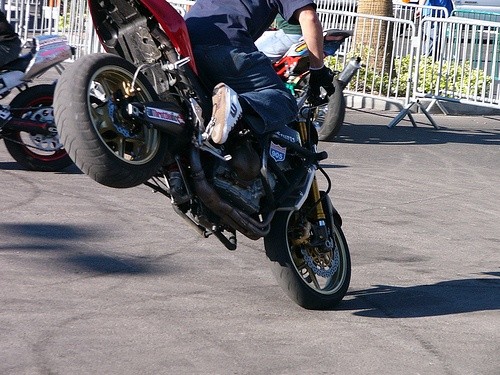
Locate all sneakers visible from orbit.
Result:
[210,83,243,145]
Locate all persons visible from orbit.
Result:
[256,14,301,60]
[181,0,336,144]
[0,12,21,68]
[416,0,454,62]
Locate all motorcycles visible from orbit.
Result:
[0,31,75,172]
[52,0,352,311]
[254,27,362,139]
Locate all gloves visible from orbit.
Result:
[307,64,337,106]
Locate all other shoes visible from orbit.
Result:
[0,108,14,129]
